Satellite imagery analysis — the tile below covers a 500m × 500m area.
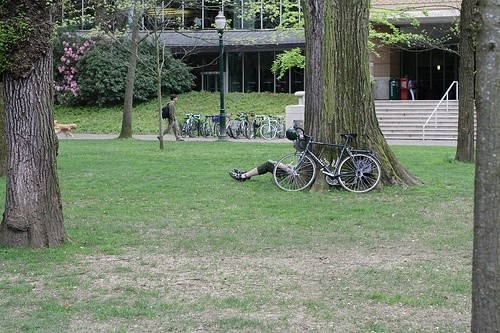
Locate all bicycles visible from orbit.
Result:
[273,127,383,194]
[181,112,285,140]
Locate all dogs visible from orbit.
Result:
[54,120,78,139]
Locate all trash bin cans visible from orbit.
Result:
[293,120,304,130]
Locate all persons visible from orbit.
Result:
[157,93,185,141]
[229,159,297,183]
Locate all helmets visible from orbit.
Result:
[286,128,297,140]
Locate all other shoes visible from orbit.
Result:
[157,137,160,140]
[176,139,184,141]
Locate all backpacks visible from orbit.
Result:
[162,103,170,119]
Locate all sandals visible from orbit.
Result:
[229,171,247,182]
[234,168,251,180]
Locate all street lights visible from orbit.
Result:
[214,10,228,140]
